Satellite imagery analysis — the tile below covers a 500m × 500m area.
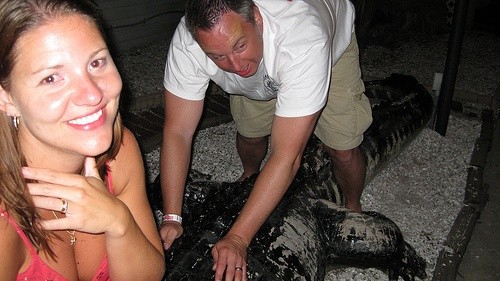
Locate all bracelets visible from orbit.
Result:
[163,214,182,224]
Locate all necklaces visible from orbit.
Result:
[158,0,373,281]
[52,211,76,245]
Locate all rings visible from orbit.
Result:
[59,198,68,213]
[235,267,242,271]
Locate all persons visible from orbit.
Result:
[0,0,166,281]
